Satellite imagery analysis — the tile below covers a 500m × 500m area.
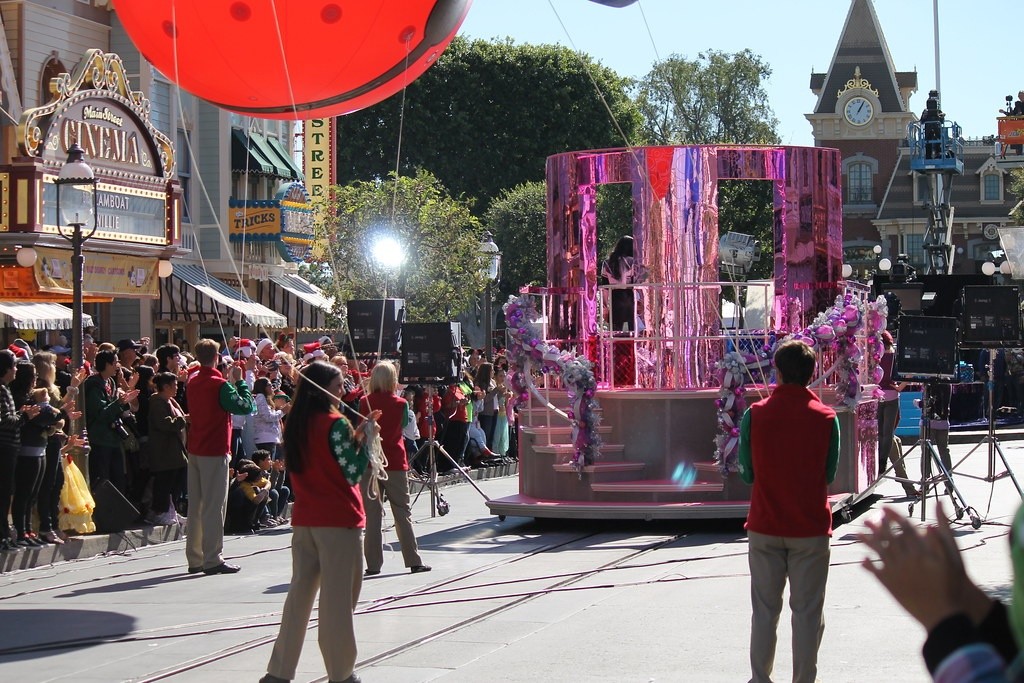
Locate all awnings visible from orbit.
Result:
[266,274,348,321]
[0,303,94,332]
[161,263,287,328]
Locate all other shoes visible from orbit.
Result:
[918,484,929,493]
[484,452,501,460]
[907,491,922,499]
[54,529,69,541]
[944,487,952,495]
[259,516,289,530]
[0,533,48,553]
[40,530,64,544]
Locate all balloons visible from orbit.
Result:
[113,0,473,123]
[158,261,173,277]
[17,248,38,267]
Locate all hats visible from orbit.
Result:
[263,360,282,372]
[48,345,71,355]
[116,338,143,352]
[235,339,258,351]
[302,353,316,363]
[318,335,332,346]
[256,339,273,357]
[312,349,325,357]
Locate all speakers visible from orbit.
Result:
[401,321,462,377]
[962,286,1019,343]
[897,316,956,374]
[345,298,406,351]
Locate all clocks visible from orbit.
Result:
[844,96,874,126]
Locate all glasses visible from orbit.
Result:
[265,383,272,387]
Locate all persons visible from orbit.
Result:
[260,361,383,683]
[179,338,252,574]
[858,494,1024,683]
[920,109,945,160]
[1000,92,1024,155]
[600,235,635,334]
[359,361,432,575]
[0,336,544,554]
[739,340,840,683]
[877,330,953,500]
[980,346,1007,421]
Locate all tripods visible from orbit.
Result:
[407,382,505,520]
[844,350,1024,531]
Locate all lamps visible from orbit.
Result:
[159,256,173,278]
[17,245,37,267]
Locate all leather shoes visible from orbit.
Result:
[364,569,381,575]
[411,564,432,573]
[328,672,361,683]
[259,673,290,683]
[188,567,204,574]
[204,561,241,576]
